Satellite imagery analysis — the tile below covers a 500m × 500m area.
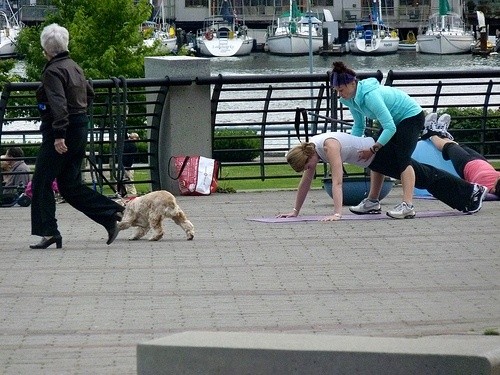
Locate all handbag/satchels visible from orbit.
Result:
[168,154,222,196]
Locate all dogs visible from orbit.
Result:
[111,189,196,242]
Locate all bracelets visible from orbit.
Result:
[333,213,341,217]
[293,208,298,213]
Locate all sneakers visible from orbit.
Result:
[462,183,488,215]
[420,113,452,137]
[349,196,383,215]
[386,201,417,219]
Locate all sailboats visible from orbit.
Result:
[196,0,256,57]
[0,0,23,57]
[140,0,178,54]
[416,0,475,55]
[264,0,324,54]
[348,0,399,54]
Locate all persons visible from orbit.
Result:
[421,112,500,198]
[274,132,487,222]
[29,24,125,249]
[0,148,33,195]
[327,62,426,219]
[117,133,140,197]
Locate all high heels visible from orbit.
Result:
[106,214,122,245]
[30,234,62,249]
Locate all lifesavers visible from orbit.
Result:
[228,31,234,39]
[205,31,213,41]
[391,31,396,37]
